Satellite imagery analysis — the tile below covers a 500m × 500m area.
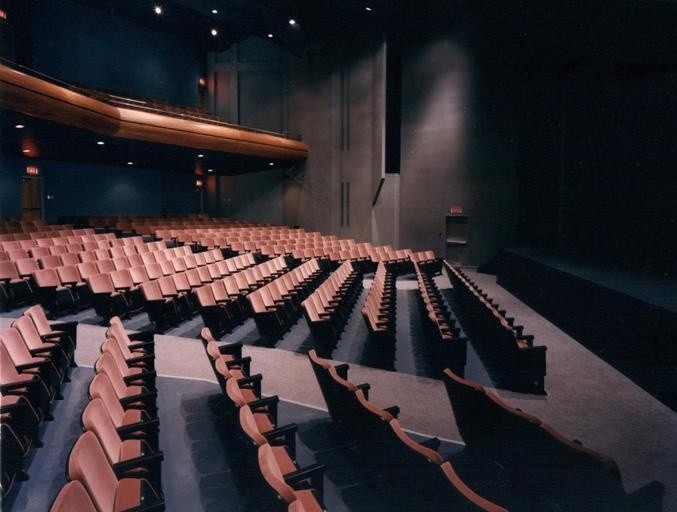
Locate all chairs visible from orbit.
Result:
[389,417,445,510]
[215,358,262,394]
[24,305,79,368]
[206,340,245,365]
[67,431,166,511]
[226,376,277,421]
[110,315,155,341]
[361,260,398,370]
[0,423,30,512]
[0,341,59,422]
[96,351,158,417]
[81,398,164,487]
[1,391,46,448]
[240,403,297,459]
[49,479,99,511]
[290,499,327,512]
[443,368,488,471]
[413,260,468,367]
[255,258,330,345]
[0,218,60,309]
[540,421,665,512]
[258,442,326,501]
[330,367,370,430]
[354,388,400,491]
[330,258,363,348]
[139,252,255,346]
[100,338,156,389]
[10,315,74,383]
[443,249,548,395]
[484,389,542,488]
[1,327,65,400]
[60,265,139,320]
[88,371,159,440]
[441,460,508,510]
[106,323,156,370]
[308,350,351,425]
